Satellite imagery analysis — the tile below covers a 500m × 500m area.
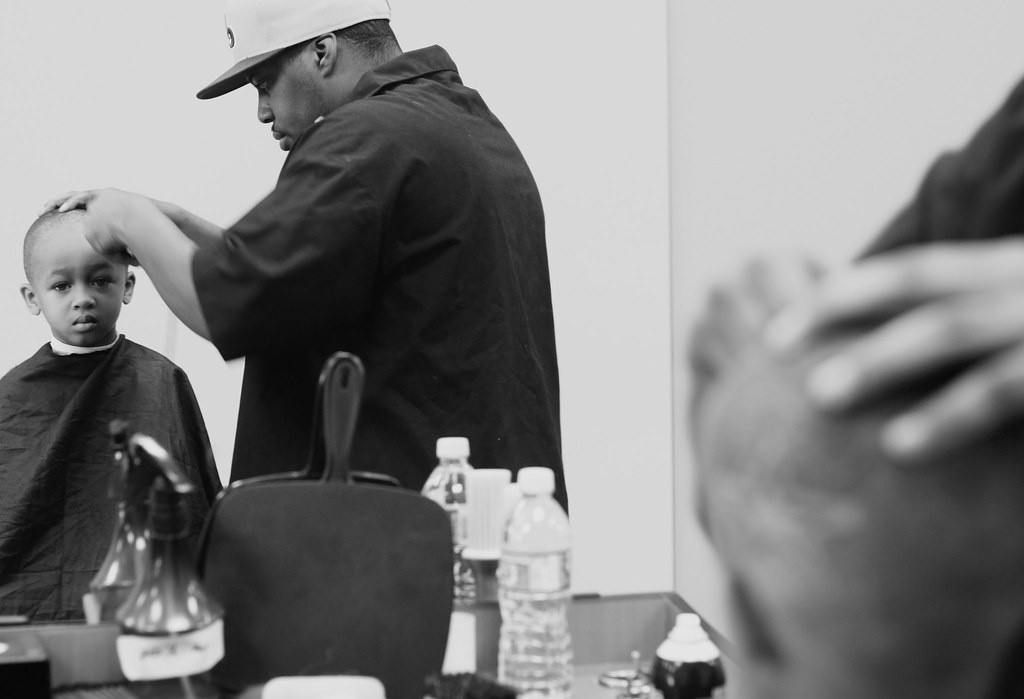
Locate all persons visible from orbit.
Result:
[0,208,221,625]
[57,0,567,510]
[687,71,1023,699]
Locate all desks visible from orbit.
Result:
[0,590,741,699]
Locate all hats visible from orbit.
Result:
[196,0,393,100]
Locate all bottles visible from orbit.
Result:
[492,466,574,699]
[418,436,490,603]
